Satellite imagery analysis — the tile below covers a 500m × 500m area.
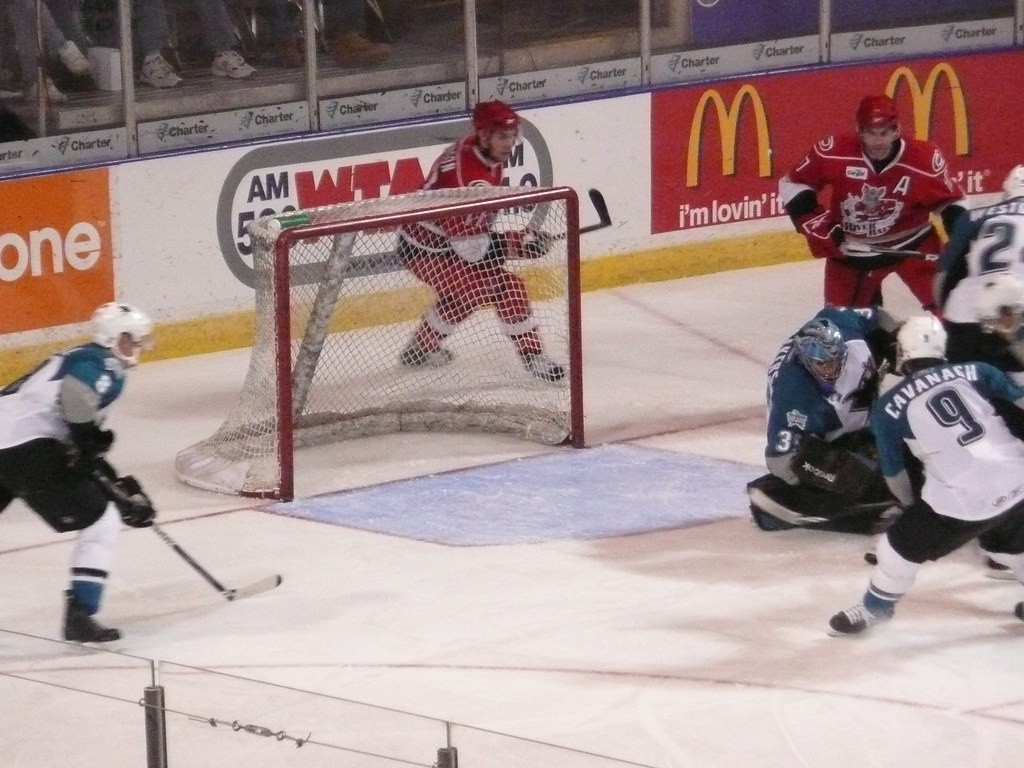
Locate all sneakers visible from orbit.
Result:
[525,355,566,389]
[209,51,260,80]
[828,604,889,639]
[985,555,1014,581]
[139,54,183,91]
[59,43,97,82]
[26,79,68,105]
[63,589,120,651]
[400,346,449,368]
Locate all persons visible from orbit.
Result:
[396,100,564,384]
[776,96,971,329]
[0,0,393,107]
[826,309,1024,637]
[935,163,1024,411]
[747,305,902,533]
[0,301,160,641]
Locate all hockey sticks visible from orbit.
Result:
[65,445,284,602]
[551,186,612,242]
[843,239,940,261]
[747,484,897,531]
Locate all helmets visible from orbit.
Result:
[976,273,1024,332]
[797,317,846,382]
[473,101,518,131]
[1002,165,1024,198]
[855,95,899,134]
[91,302,153,367]
[898,315,947,375]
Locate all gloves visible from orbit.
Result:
[505,229,551,259]
[115,475,155,528]
[796,208,848,259]
[68,418,115,453]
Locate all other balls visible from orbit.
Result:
[864,552,878,565]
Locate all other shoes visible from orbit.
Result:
[276,39,302,67]
[332,33,392,67]
